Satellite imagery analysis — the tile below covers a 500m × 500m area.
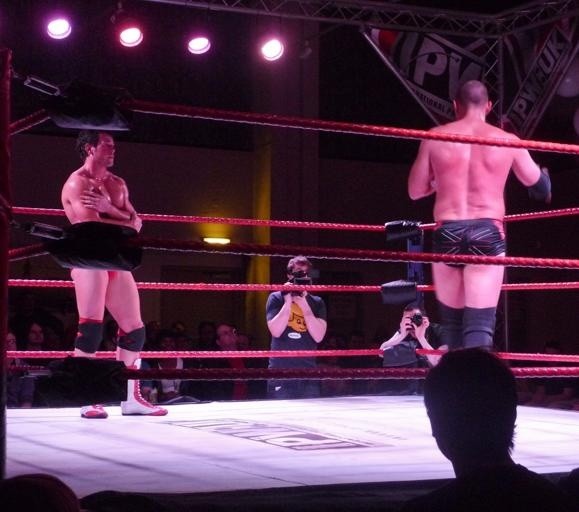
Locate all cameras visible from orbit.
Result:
[411,311,424,331]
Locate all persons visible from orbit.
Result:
[261,255,330,397]
[61,128,170,418]
[517,344,574,407]
[406,81,554,355]
[314,331,385,396]
[0,472,88,511]
[101,318,268,401]
[2,322,54,410]
[405,347,578,510]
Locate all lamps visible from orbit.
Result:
[40,0,288,66]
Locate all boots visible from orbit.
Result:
[120,357,167,416]
[81,404,107,418]
